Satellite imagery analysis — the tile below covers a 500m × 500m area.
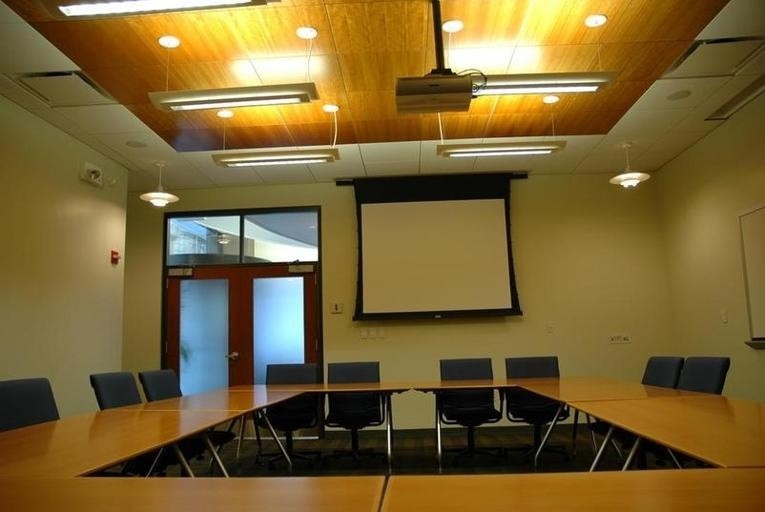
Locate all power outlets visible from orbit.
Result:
[608,334,632,344]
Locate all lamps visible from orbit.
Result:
[52,0,283,24]
[608,141,650,190]
[147,26,318,114]
[139,160,180,207]
[436,95,567,159]
[440,15,616,98]
[211,104,340,169]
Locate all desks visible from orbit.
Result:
[379,466,765,512]
[569,396,764,467]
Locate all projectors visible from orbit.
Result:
[395,67,488,113]
